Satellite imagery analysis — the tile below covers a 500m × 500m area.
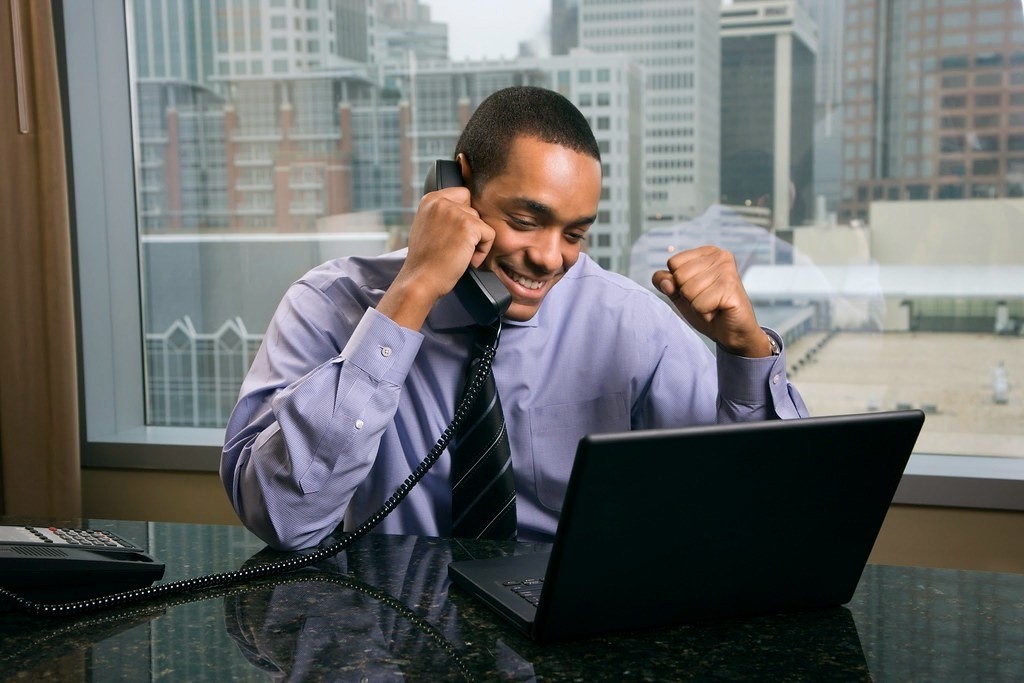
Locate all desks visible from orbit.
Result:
[0,513,1024,683]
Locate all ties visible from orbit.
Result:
[448,324,517,540]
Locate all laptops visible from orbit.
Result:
[445,408,924,646]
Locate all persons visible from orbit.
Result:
[629,154,882,381]
[218,86,808,552]
[224,532,539,683]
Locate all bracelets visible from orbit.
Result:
[767,334,781,356]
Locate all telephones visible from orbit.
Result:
[422,160,514,327]
